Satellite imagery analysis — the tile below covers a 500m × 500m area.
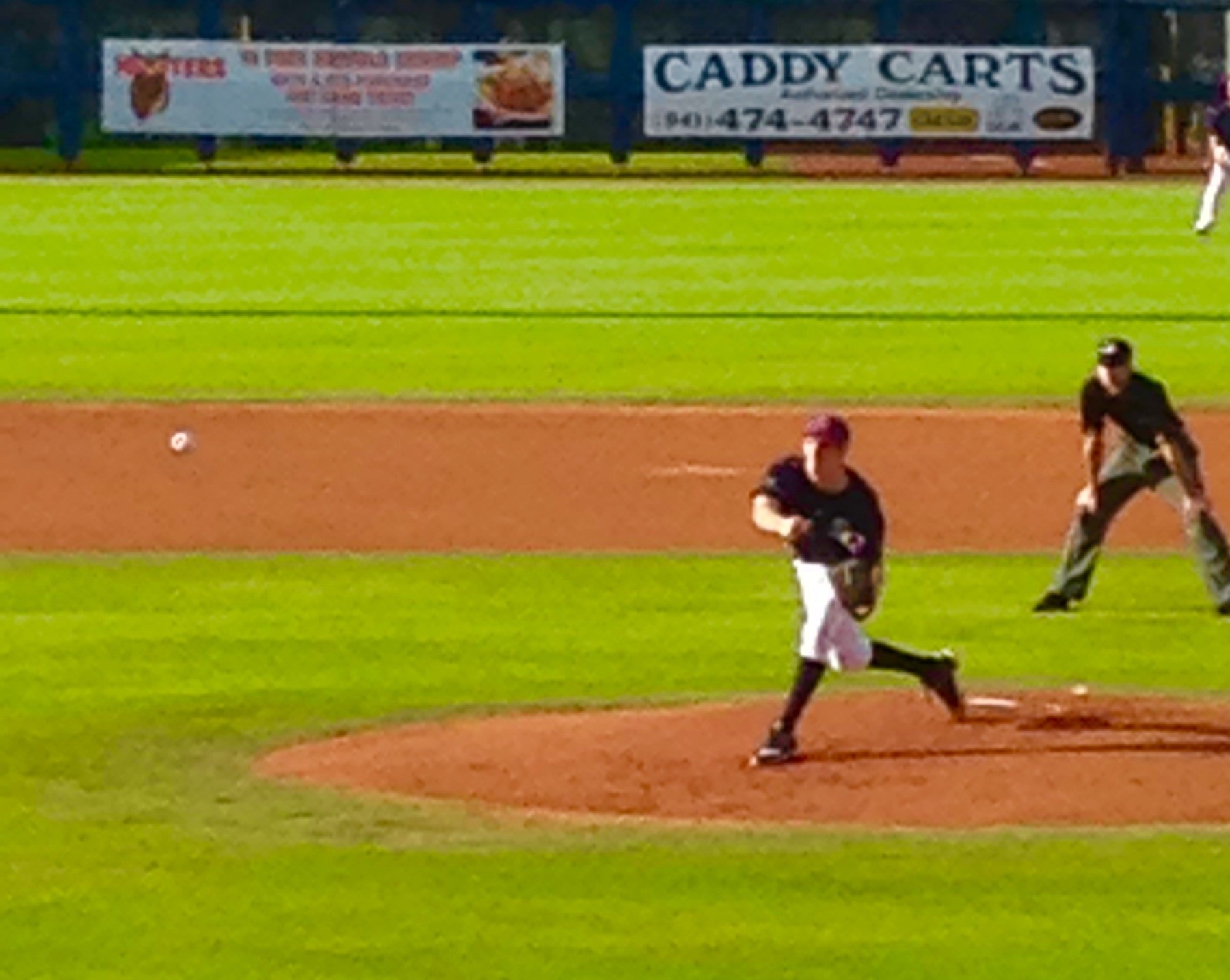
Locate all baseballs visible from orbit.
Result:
[169,432,195,455]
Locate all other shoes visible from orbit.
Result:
[1218,600,1230,613]
[1032,593,1069,611]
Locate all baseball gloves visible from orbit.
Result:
[827,560,877,621]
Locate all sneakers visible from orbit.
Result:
[929,648,965,721]
[755,726,797,763]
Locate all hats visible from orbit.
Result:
[1095,338,1132,365]
[802,413,848,442]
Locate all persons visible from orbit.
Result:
[1192,75,1230,233]
[747,413,967,769]
[1030,338,1230,617]
